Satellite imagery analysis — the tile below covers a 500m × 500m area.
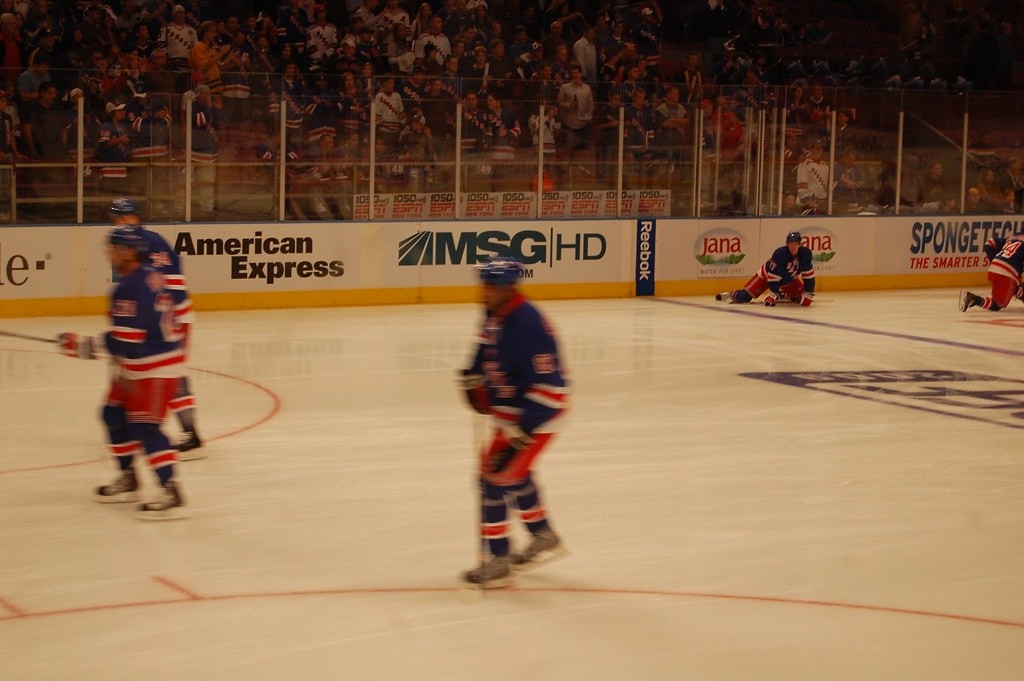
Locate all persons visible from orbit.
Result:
[56,227,188,514]
[715,230,816,308]
[105,197,204,454]
[0,0,1024,215]
[962,230,1024,312]
[454,255,574,585]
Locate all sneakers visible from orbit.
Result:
[462,557,515,588]
[95,467,141,502]
[170,433,205,459]
[958,289,975,312]
[716,291,729,301]
[136,480,191,521]
[507,525,570,571]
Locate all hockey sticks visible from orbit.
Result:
[472,411,518,592]
[0,329,59,343]
[730,298,831,304]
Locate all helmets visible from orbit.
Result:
[107,198,143,215]
[785,230,802,243]
[479,256,523,285]
[108,226,146,246]
